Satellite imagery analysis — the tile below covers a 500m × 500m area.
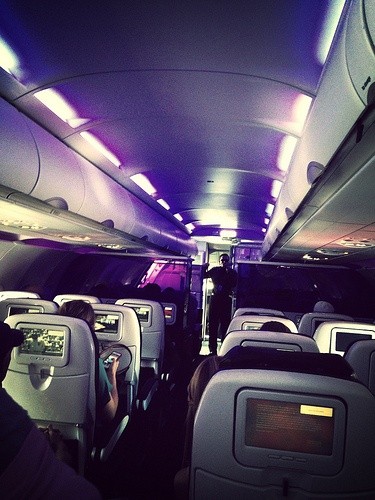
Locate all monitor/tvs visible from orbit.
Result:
[162,305,174,323]
[242,323,263,330]
[331,328,375,358]
[14,323,70,367]
[6,304,45,317]
[124,304,152,327]
[235,390,347,475]
[312,318,338,337]
[93,310,123,342]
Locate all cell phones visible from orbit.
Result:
[104,351,122,364]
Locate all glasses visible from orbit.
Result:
[221,259,229,261]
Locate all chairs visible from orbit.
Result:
[0,290,178,479]
[187,308,375,500]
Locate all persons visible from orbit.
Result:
[0,319,57,500]
[20,281,198,334]
[173,357,238,492]
[60,299,121,420]
[201,254,239,355]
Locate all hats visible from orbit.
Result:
[313,301,335,313]
[0,321,24,348]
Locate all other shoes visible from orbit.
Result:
[208,351,217,356]
